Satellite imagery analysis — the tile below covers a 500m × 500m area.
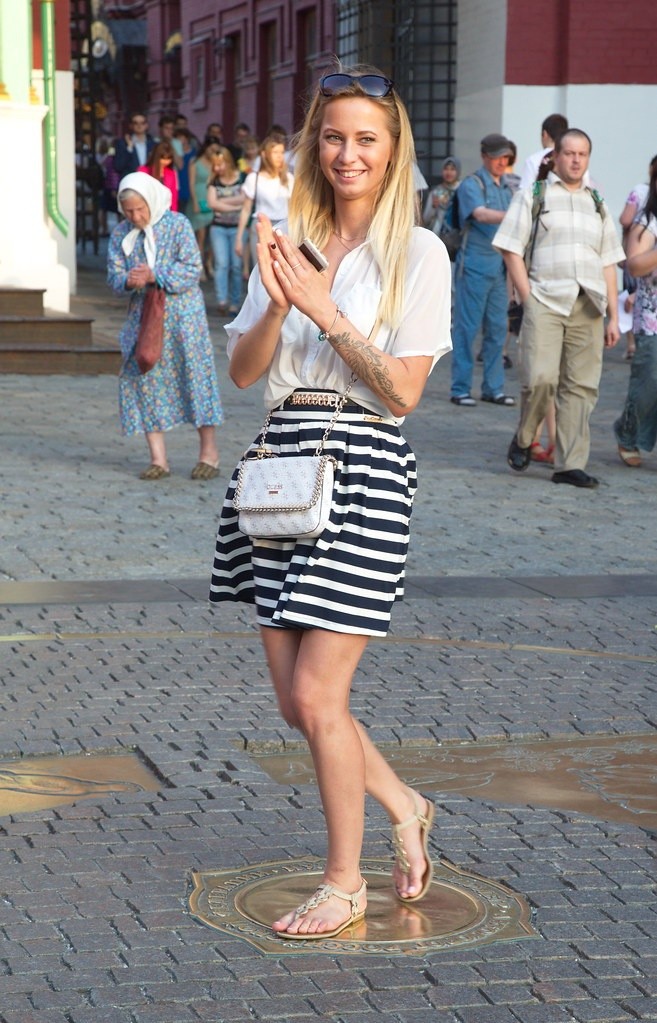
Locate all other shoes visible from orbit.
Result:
[228,310,238,319]
[504,355,512,369]
[218,304,228,311]
[477,350,483,361]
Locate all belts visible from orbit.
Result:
[211,219,239,228]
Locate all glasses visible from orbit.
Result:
[132,120,145,124]
[319,73,394,98]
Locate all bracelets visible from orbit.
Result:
[317,305,341,342]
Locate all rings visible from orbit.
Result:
[292,263,300,269]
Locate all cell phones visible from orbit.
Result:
[297,238,329,273]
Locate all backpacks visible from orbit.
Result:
[437,172,517,262]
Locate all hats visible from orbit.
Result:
[481,134,515,158]
[442,157,461,180]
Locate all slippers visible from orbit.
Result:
[140,463,170,480]
[191,462,220,481]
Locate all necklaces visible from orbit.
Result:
[336,232,354,251]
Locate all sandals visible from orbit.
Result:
[617,441,641,467]
[530,442,555,464]
[450,395,476,407]
[480,395,515,406]
[390,786,434,904]
[621,343,635,360]
[276,877,368,939]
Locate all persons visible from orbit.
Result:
[413,113,657,489]
[93,111,298,315]
[104,172,224,481]
[209,69,455,939]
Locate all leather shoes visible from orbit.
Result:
[551,469,599,487]
[507,434,532,472]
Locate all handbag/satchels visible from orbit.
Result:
[508,300,524,336]
[233,454,338,539]
[622,262,638,294]
[135,280,166,375]
